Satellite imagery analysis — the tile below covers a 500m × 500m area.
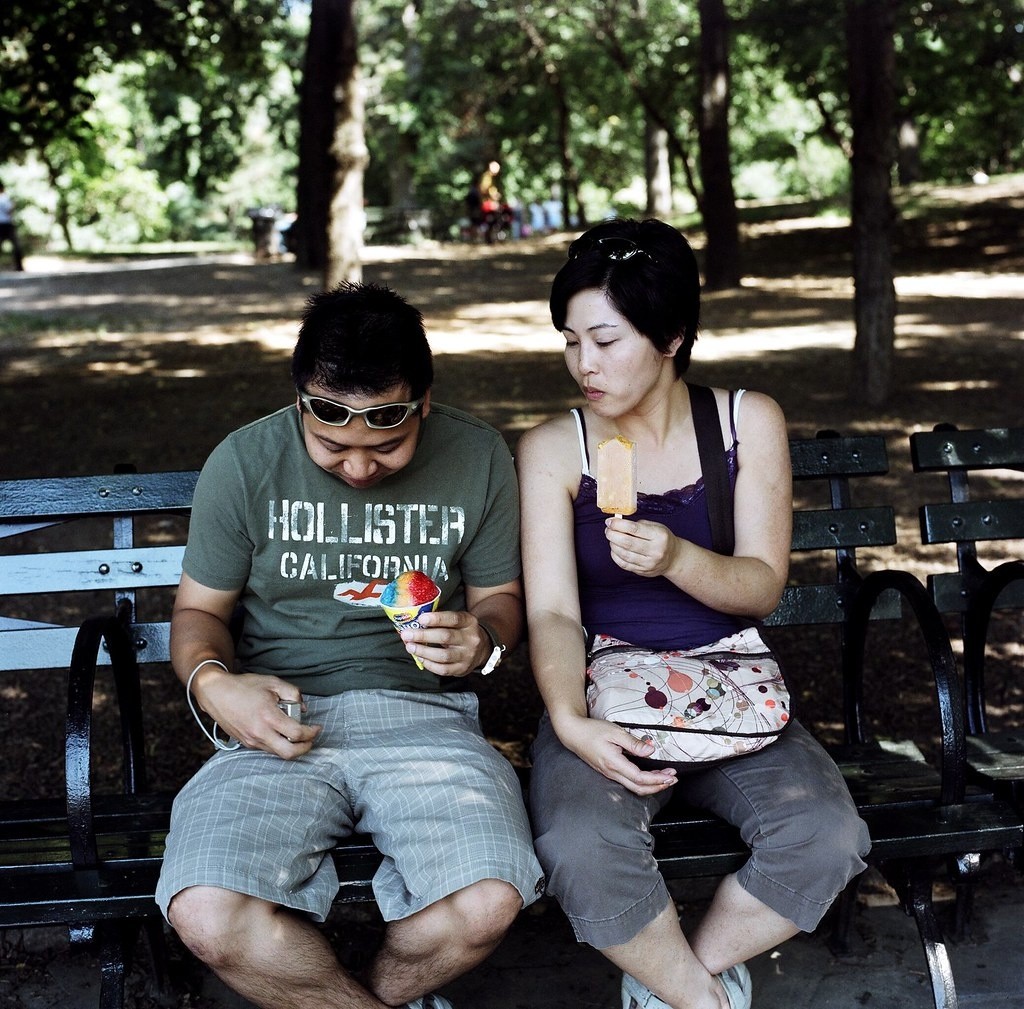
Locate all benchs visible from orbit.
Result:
[0,422,1024,1009]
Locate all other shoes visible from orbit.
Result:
[406,991,453,1009]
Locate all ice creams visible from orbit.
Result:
[380,570,440,607]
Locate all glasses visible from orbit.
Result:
[567,234,665,267]
[294,378,427,429]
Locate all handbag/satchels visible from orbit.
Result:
[584,625,789,762]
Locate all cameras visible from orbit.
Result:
[277,700,301,744]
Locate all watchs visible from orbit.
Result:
[474,617,507,676]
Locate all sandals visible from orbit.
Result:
[621,971,673,1009]
[716,959,753,1009]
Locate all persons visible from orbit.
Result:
[153,284,547,1009]
[479,161,502,245]
[517,217,872,1009]
[0,181,26,271]
